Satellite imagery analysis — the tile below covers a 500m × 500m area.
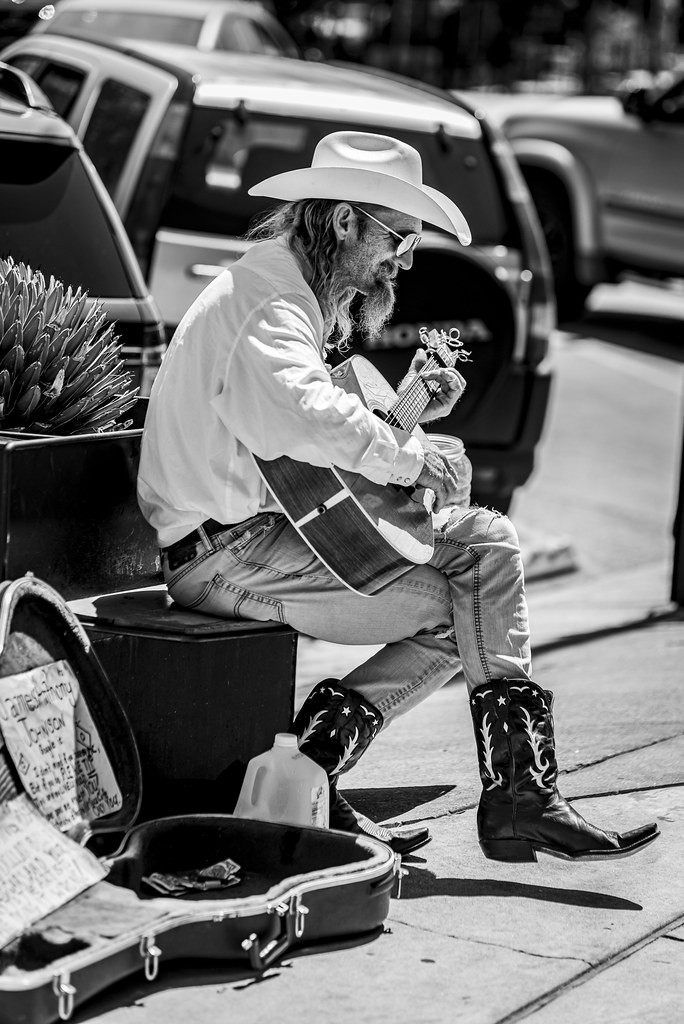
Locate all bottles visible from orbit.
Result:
[229,733,331,833]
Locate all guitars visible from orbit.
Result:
[250,327,474,597]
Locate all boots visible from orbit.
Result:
[470,678,661,863]
[292,677,433,856]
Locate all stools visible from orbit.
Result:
[67,584,299,825]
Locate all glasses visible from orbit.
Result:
[350,204,422,257]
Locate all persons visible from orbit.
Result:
[135,131,660,864]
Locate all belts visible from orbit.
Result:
[161,518,237,555]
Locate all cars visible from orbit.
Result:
[443,53,683,330]
[0,59,168,422]
[33,0,306,61]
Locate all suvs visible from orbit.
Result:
[0,31,558,515]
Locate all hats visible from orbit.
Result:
[248,131,472,247]
